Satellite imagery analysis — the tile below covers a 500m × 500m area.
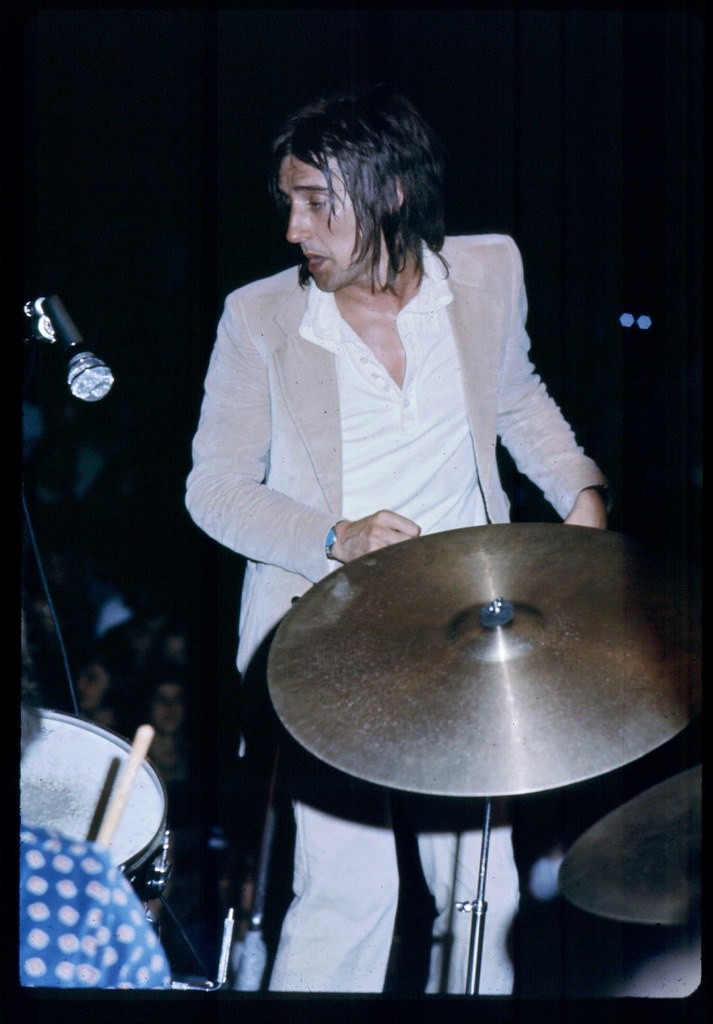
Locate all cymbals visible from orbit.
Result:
[556,759,701,929]
[263,519,710,801]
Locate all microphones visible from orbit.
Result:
[41,294,114,402]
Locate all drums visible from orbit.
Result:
[18,702,172,925]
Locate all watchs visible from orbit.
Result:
[325,520,342,559]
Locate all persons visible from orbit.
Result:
[184,93,612,994]
[19,576,182,991]
